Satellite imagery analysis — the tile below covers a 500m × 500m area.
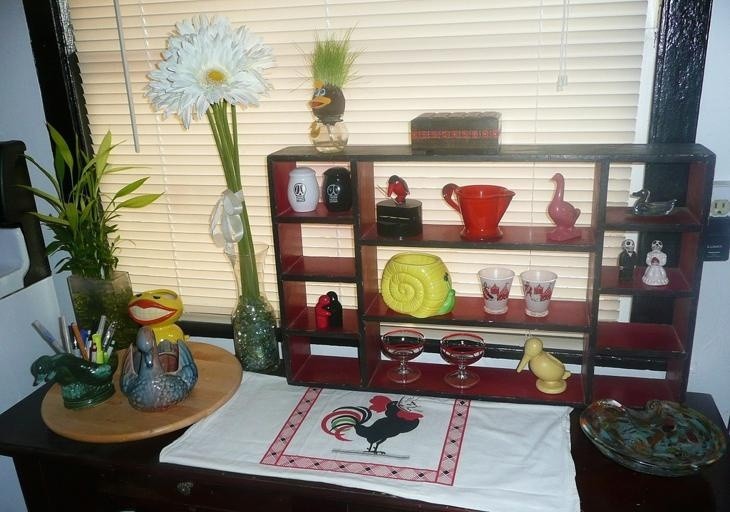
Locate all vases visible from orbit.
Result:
[225,243,284,373]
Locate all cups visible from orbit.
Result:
[439,332,485,390]
[475,267,515,316]
[380,328,425,387]
[518,268,559,319]
[441,184,516,240]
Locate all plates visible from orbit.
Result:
[578,397,728,478]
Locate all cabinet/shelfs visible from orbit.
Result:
[0,0,76,512]
[267,144,715,408]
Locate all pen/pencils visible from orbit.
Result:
[85,314,119,365]
[31,319,66,354]
[71,321,89,362]
[72,336,81,359]
[59,315,88,360]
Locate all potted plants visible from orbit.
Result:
[11,120,164,351]
[298,25,367,152]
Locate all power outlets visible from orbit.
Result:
[709,180,729,218]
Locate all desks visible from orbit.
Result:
[0,377,730,512]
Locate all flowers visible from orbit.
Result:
[142,13,275,293]
